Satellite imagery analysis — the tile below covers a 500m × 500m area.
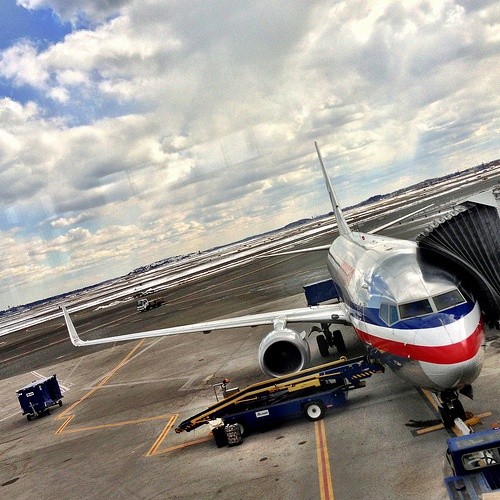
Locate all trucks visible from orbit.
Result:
[136,297,164,312]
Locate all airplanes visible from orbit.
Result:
[57,142,500,437]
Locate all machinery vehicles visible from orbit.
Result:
[443,429,500,500]
